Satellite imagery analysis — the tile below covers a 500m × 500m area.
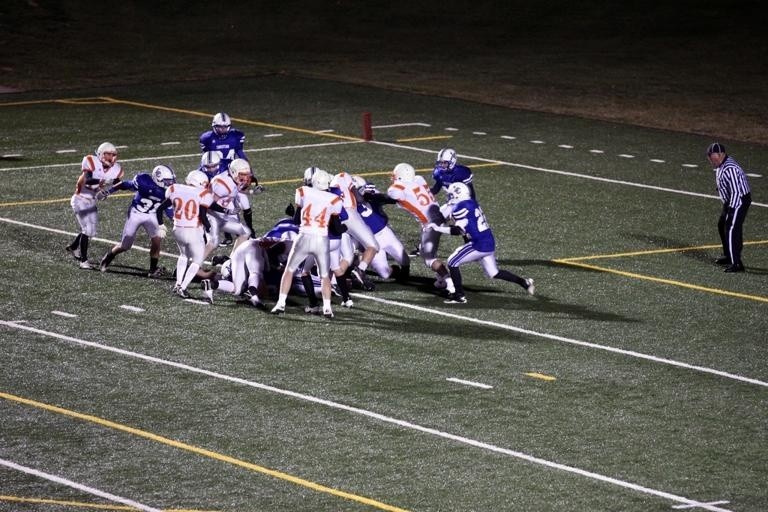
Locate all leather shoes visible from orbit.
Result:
[714,256,732,265]
[724,261,745,272]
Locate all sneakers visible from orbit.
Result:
[147,268,173,279]
[304,304,336,319]
[434,272,469,305]
[66,245,81,261]
[212,254,231,266]
[330,283,342,297]
[350,266,376,292]
[79,259,94,270]
[340,299,354,309]
[243,287,286,317]
[200,278,214,305]
[408,248,421,257]
[219,238,233,247]
[99,251,113,272]
[172,284,190,298]
[524,278,536,296]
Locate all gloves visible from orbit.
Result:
[252,185,265,195]
[157,223,169,240]
[422,222,451,235]
[99,177,114,188]
[94,189,111,203]
[224,208,242,216]
[285,202,295,217]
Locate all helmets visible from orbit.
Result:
[392,163,415,183]
[185,169,209,189]
[94,141,118,168]
[435,148,457,174]
[706,142,726,154]
[228,157,252,185]
[303,164,366,193]
[211,112,232,141]
[152,164,176,191]
[200,149,221,173]
[447,181,471,204]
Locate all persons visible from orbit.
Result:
[705,143,751,273]
[427,147,476,244]
[426,181,535,303]
[96,165,175,278]
[63,141,123,269]
[381,162,456,298]
[200,112,261,246]
